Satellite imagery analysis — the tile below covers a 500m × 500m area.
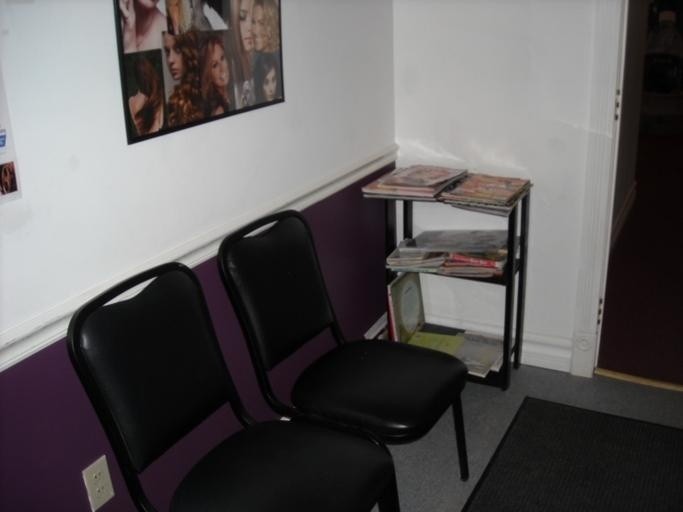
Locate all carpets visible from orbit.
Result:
[463,397,683,510]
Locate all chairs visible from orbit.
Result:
[65,259,402,511]
[216,209,468,483]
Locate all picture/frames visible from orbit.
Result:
[111,0,286,145]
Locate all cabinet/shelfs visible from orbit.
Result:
[383,175,532,391]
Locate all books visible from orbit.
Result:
[360,165,531,382]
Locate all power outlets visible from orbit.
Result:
[81,454,116,511]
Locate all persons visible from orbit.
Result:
[120,1,283,137]
